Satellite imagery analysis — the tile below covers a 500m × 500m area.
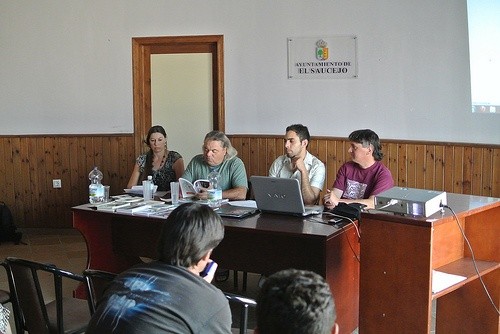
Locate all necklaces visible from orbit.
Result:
[322,129,393,221]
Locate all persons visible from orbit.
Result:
[86,202,233,334]
[268,124,325,206]
[253,269,339,334]
[127,125,186,194]
[177,130,249,202]
[0,303,13,334]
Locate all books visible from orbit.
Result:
[92,178,257,218]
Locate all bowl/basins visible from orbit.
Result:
[132,185,158,193]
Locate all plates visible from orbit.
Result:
[124,188,143,193]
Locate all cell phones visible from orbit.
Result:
[200,262,214,276]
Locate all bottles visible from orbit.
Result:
[88,165,105,205]
[206,167,222,210]
[148,175,154,199]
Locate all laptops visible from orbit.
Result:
[250,175,327,217]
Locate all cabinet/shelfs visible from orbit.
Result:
[359,191,500,334]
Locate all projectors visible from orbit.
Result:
[375,187,448,219]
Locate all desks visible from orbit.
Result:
[72,201,360,334]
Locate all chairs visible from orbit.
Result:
[223,292,258,334]
[83,269,119,314]
[0,257,92,334]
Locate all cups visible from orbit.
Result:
[103,185,110,203]
[170,182,179,205]
[142,180,152,201]
